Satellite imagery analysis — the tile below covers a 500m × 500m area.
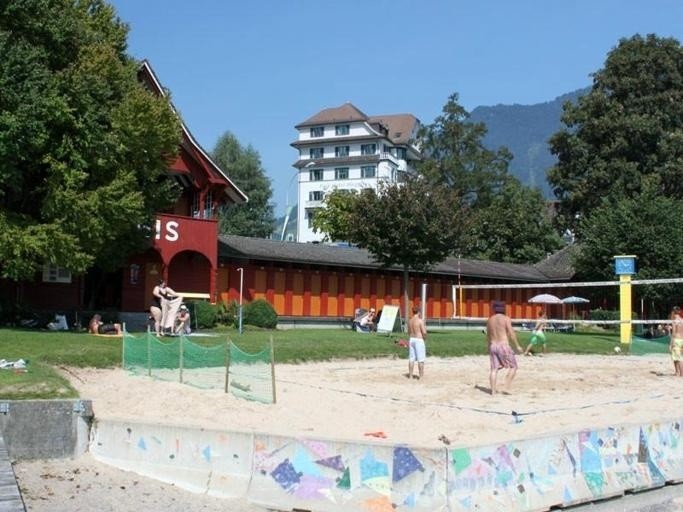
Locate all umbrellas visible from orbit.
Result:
[561,296,590,334]
[527,294,562,327]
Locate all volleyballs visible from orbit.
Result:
[615,346,621,354]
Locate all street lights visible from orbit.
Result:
[279,160,317,242]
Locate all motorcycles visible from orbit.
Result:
[5,301,43,331]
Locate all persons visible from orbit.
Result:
[149,279,175,338]
[485,302,523,396]
[89,314,122,335]
[357,308,375,332]
[524,310,547,356]
[405,305,427,382]
[172,304,193,335]
[669,306,682,377]
